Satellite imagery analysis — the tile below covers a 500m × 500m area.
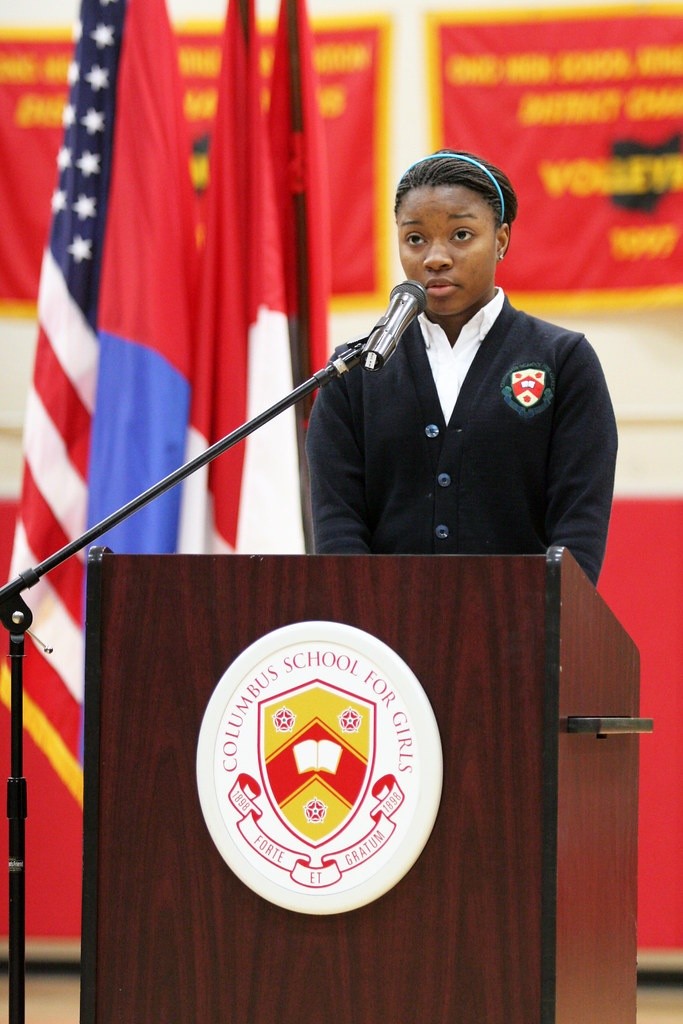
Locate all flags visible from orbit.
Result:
[0,0,331,805]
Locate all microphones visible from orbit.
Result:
[359,280,427,372]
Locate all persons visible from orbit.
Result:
[303,148,622,591]
